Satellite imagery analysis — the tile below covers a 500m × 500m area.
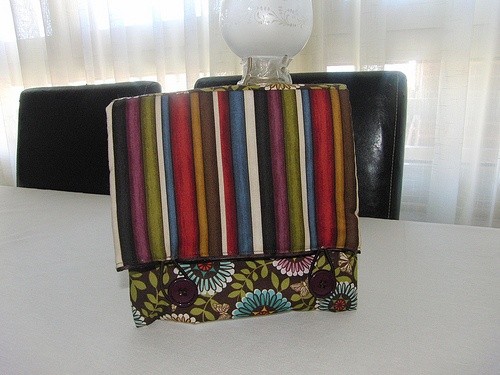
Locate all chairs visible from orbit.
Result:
[15,81,162,195]
[194,71,408,220]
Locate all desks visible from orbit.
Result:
[0,185,500,375]
